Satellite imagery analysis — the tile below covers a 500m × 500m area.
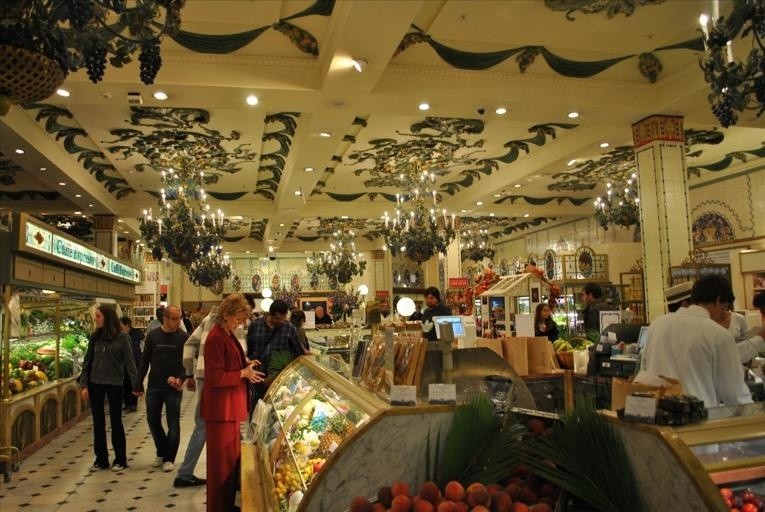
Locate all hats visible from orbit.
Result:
[666,282,693,306]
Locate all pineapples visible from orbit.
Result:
[319,411,344,456]
[341,405,364,439]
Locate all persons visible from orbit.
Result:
[715,308,752,370]
[147,308,163,331]
[179,310,193,334]
[174,304,248,488]
[677,301,689,313]
[420,287,453,340]
[290,310,310,350]
[248,300,305,424]
[315,307,332,324]
[582,283,612,333]
[753,292,765,339]
[80,305,144,471]
[191,302,206,330]
[410,304,423,321]
[131,305,190,472]
[118,317,149,413]
[251,312,260,321]
[200,294,265,512]
[534,303,558,342]
[640,274,754,410]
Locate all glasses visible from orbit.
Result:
[168,316,184,321]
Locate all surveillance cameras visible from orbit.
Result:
[478,109,485,114]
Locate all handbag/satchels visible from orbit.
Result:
[76,341,94,384]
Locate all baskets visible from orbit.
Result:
[556,337,584,368]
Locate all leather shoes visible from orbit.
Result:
[174,476,207,487]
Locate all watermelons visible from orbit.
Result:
[48,360,70,378]
[41,354,55,365]
[61,359,80,374]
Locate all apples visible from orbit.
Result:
[313,460,324,474]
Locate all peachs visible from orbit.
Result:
[493,490,512,512]
[410,495,420,505]
[446,481,464,501]
[472,505,490,512]
[392,495,411,512]
[486,485,505,495]
[456,501,468,512]
[507,483,521,496]
[419,482,441,505]
[391,481,411,498]
[373,502,385,512]
[437,500,457,512]
[530,503,549,511]
[378,486,394,509]
[466,483,489,506]
[414,499,434,512]
[350,495,374,512]
[513,502,529,512]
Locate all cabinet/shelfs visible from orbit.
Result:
[130,281,160,329]
[241,356,511,512]
[0,370,91,467]
[473,273,550,338]
[618,269,645,325]
[597,404,764,510]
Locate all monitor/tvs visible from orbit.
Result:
[635,326,648,351]
[433,316,467,339]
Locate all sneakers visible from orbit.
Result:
[163,462,174,473]
[90,462,103,471]
[112,464,125,471]
[152,457,164,466]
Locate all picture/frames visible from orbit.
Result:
[394,287,428,313]
[298,292,345,325]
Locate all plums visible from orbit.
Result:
[752,496,765,508]
[725,497,737,509]
[720,488,732,500]
[731,508,740,512]
[743,503,757,512]
[741,491,755,503]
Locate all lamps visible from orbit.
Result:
[136,160,225,269]
[397,297,415,337]
[358,285,369,310]
[188,245,230,289]
[698,1,765,127]
[594,169,640,230]
[381,171,456,266]
[261,288,274,312]
[306,226,368,285]
